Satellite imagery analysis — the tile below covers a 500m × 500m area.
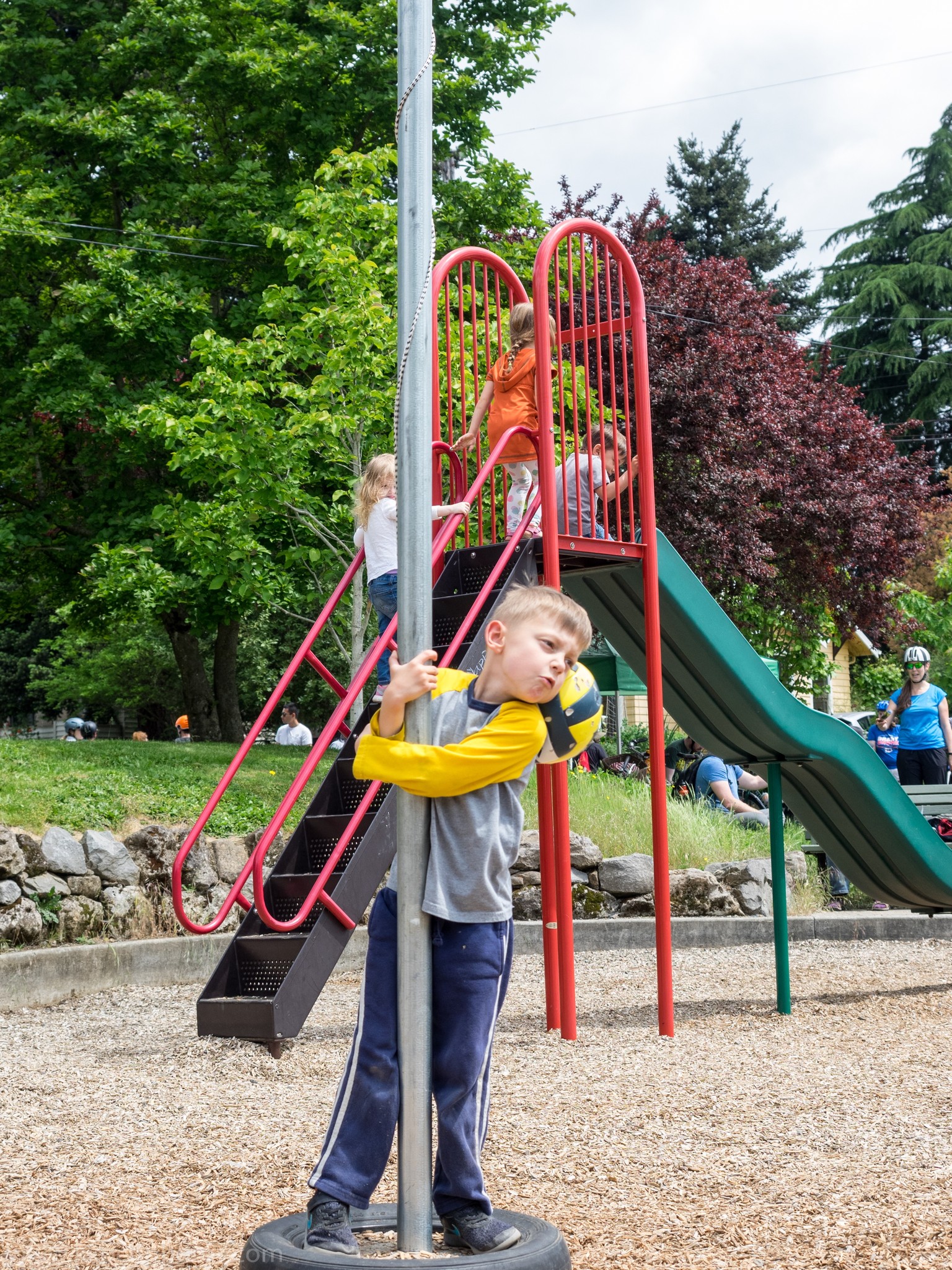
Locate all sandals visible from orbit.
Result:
[503,532,514,542]
[827,895,845,911]
[872,900,889,910]
[522,526,542,538]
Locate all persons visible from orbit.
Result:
[303,584,593,1256]
[572,731,610,777]
[79,721,100,741]
[59,718,84,742]
[133,731,148,742]
[554,422,640,542]
[824,724,890,911]
[451,300,555,542]
[661,735,705,804]
[696,752,788,832]
[175,715,192,744]
[874,645,952,842]
[275,702,313,747]
[866,700,901,785]
[353,452,399,692]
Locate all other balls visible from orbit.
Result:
[535,660,604,764]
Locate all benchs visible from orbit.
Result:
[799,785,952,909]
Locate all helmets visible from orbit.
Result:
[175,715,189,730]
[64,717,84,733]
[904,646,930,663]
[536,661,604,765]
[81,721,97,736]
[876,700,889,710]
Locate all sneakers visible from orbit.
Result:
[304,1189,360,1259]
[439,1201,521,1254]
[372,685,388,703]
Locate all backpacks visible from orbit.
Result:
[577,744,598,774]
[671,754,737,806]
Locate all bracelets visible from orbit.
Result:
[948,753,952,755]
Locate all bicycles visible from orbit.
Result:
[601,737,766,810]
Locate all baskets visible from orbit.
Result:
[602,754,648,784]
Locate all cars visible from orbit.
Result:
[831,711,877,739]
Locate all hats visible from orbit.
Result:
[592,729,606,741]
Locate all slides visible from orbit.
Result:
[555,523,952,911]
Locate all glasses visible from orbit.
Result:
[281,712,292,717]
[906,661,926,669]
